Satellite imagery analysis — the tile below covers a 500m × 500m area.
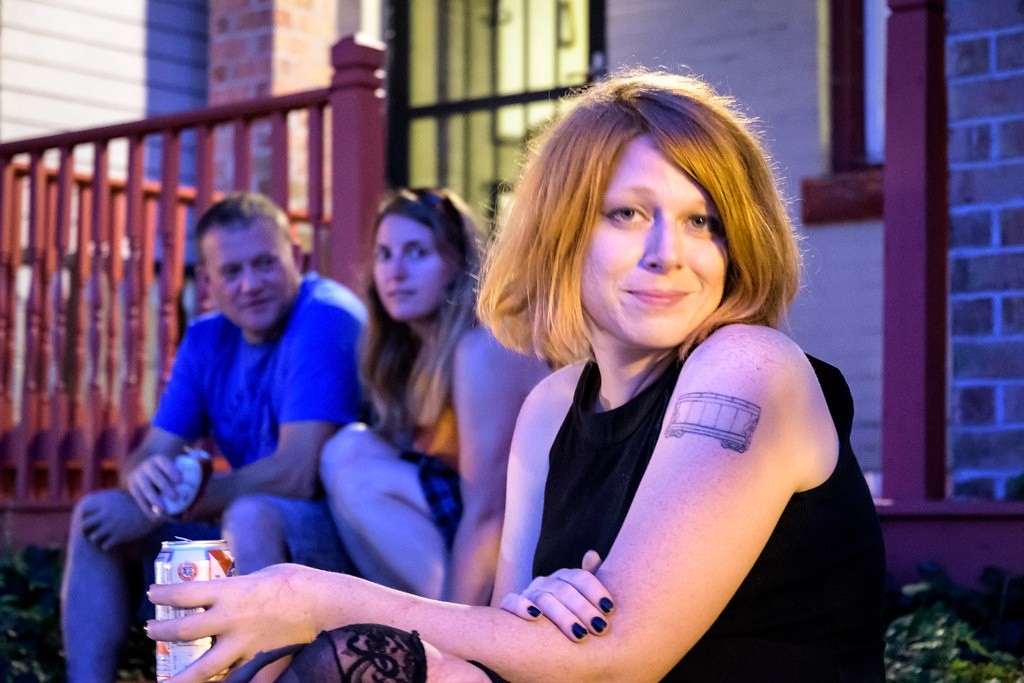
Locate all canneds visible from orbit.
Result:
[151,445,212,523]
[154,536,235,683]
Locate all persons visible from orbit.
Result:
[54,194,370,683]
[318,186,553,608]
[142,67,892,683]
